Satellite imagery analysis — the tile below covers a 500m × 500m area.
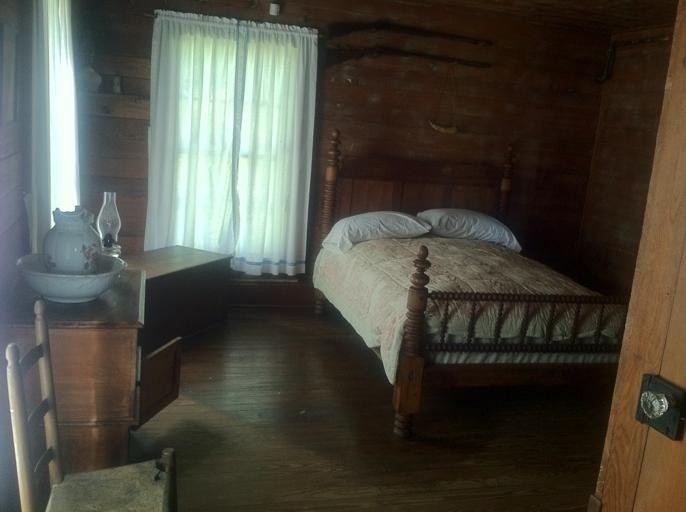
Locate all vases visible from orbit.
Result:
[97,192,127,269]
[43,205,101,275]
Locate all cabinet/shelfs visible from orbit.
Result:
[0,293,183,473]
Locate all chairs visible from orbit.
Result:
[6,298,176,512]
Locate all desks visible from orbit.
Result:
[120,245,233,326]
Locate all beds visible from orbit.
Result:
[313,128,629,438]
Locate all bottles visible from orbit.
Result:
[41,191,121,275]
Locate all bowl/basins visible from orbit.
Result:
[18,253,125,304]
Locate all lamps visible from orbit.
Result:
[269,0,281,16]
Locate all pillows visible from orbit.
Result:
[322,211,433,257]
[417,208,522,253]
